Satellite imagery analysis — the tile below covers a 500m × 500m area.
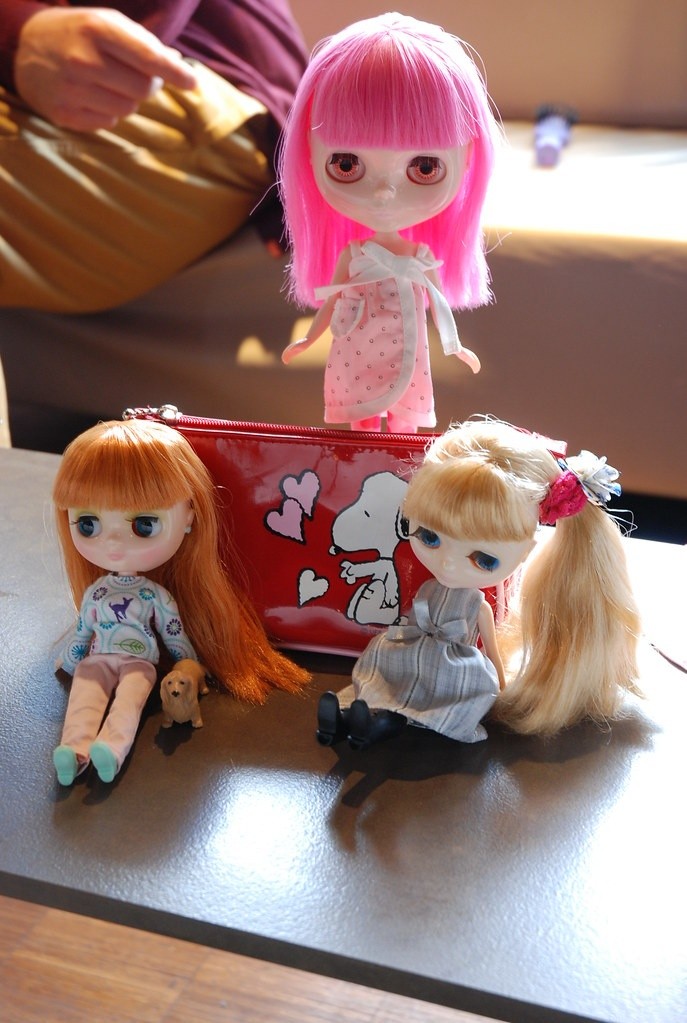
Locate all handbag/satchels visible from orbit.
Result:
[122,405,511,660]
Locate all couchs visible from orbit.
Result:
[0,0,687,500]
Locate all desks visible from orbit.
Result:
[0,447,687,1023]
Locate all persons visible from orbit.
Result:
[52,419,311,787]
[272,14,497,434]
[315,418,642,748]
[0,0,310,450]
